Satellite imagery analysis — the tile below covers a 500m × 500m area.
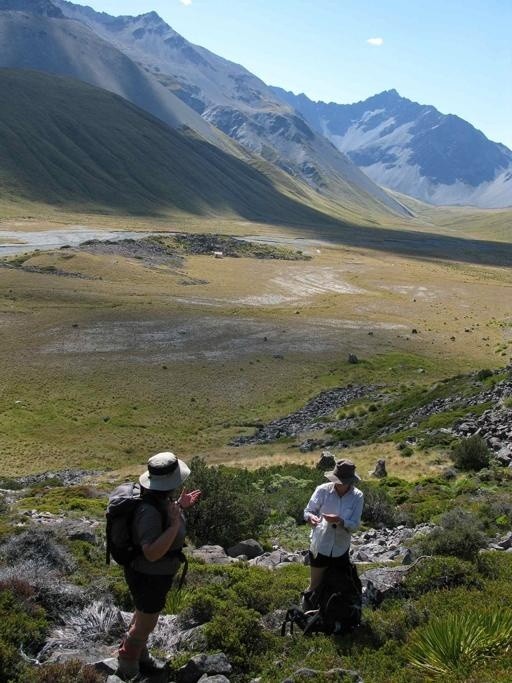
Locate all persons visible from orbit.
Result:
[302,459,364,611]
[120,452,201,683]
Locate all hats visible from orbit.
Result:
[324,460,362,484]
[139,452,191,491]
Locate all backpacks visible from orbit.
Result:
[105,481,144,568]
[281,578,362,635]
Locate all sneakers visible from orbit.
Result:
[116,651,168,683]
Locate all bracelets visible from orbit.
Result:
[175,501,184,511]
